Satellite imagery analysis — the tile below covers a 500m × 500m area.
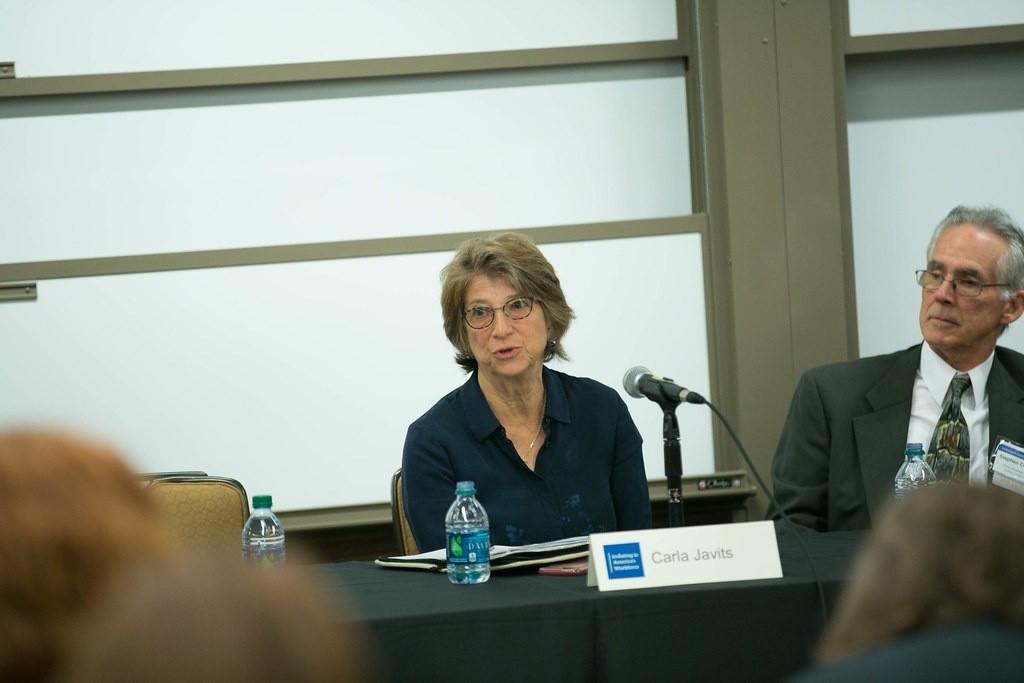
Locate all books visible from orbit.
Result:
[375,535,589,573]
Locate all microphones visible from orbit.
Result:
[624,366,706,404]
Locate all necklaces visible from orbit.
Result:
[521,384,547,463]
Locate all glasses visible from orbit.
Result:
[915,269,1013,298]
[462,295,537,330]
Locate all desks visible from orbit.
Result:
[307,529,870,683]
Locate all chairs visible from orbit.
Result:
[139,472,249,588]
[391,470,418,557]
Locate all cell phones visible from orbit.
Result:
[537,558,589,576]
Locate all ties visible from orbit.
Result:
[927,373,969,486]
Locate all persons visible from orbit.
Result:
[400,231,651,554]
[800,482,1024,683]
[766,205,1024,534]
[0,433,365,683]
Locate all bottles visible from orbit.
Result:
[895,444,936,502]
[241,496,284,578]
[446,481,491,584]
